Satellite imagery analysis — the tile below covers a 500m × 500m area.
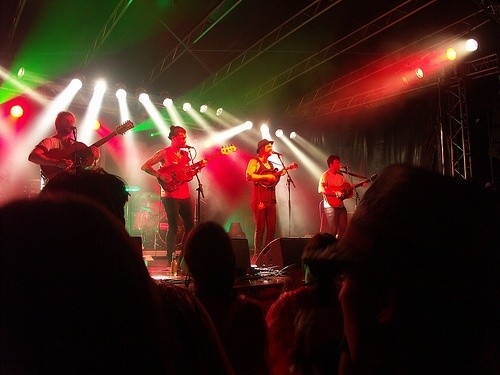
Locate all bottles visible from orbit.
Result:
[172,260,177,276]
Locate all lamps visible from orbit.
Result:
[210,102,223,116]
[135,87,148,102]
[176,95,191,112]
[115,82,127,98]
[227,222,246,239]
[160,90,173,108]
[400,29,488,85]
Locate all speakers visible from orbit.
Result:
[180,238,252,280]
[254,237,313,272]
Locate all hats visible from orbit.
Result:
[257,139,274,154]
[335,162,500,288]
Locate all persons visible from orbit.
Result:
[0,165,500,375]
[141,125,206,267]
[28,112,100,191]
[318,154,356,240]
[246,139,286,265]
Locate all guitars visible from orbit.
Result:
[157,143,237,191]
[324,173,377,209]
[40,120,135,182]
[258,162,298,189]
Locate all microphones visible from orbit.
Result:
[340,165,351,169]
[69,126,76,130]
[272,151,283,156]
[184,145,194,148]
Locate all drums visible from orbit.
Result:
[156,214,185,248]
